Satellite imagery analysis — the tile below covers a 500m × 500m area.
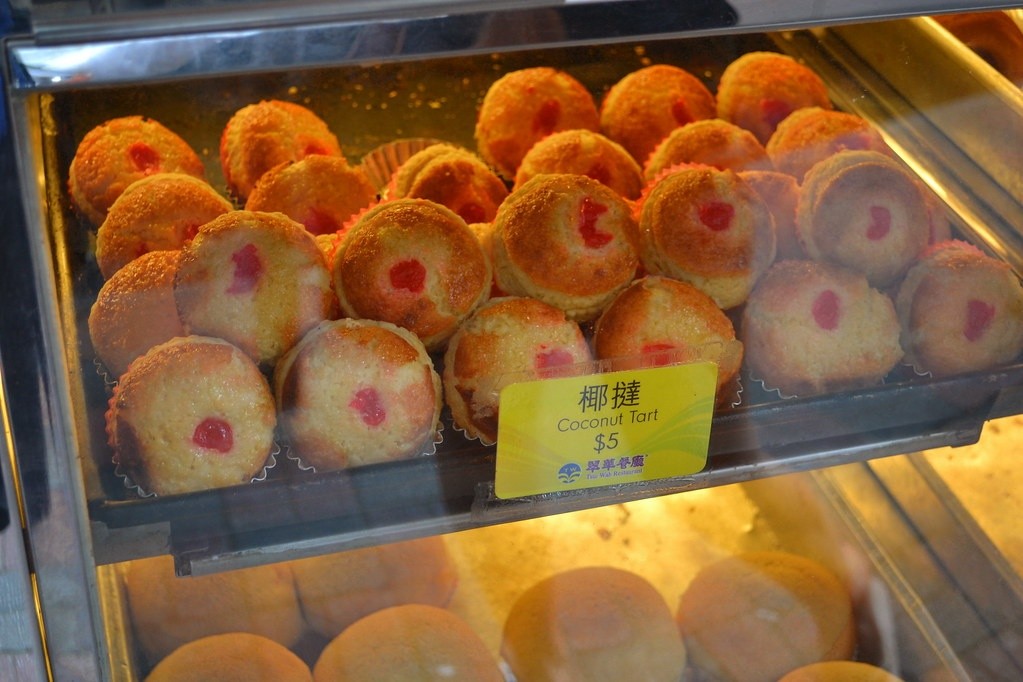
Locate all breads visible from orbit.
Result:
[67,50,1023,493]
[142,550,903,682]
